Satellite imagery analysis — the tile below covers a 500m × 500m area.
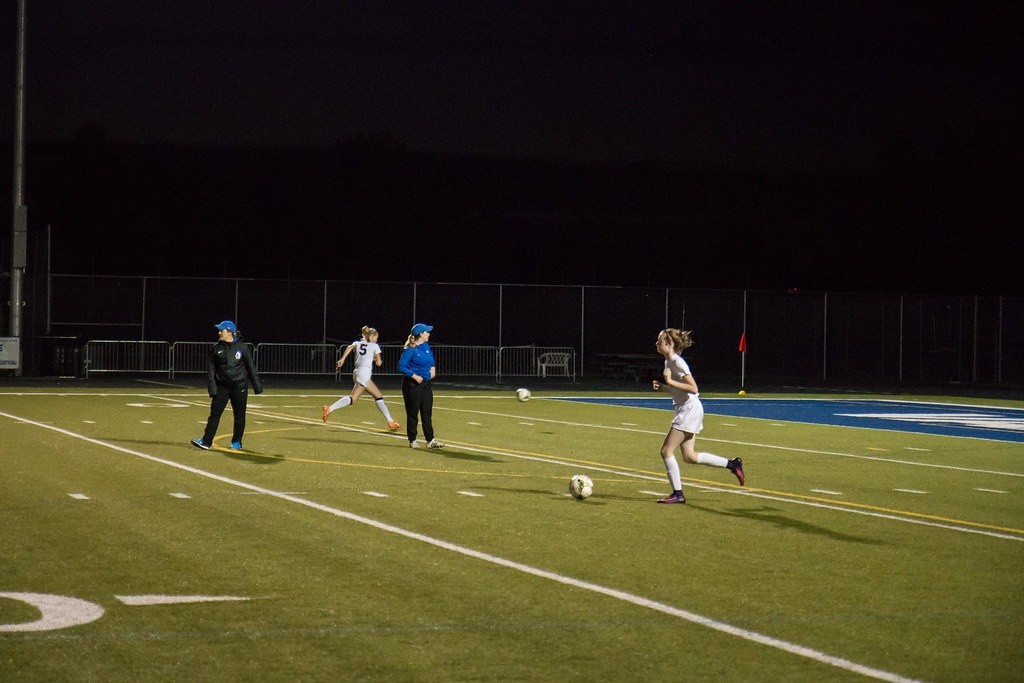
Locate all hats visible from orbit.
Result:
[410,323,433,337]
[214,321,237,333]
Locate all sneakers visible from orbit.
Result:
[191,437,211,450]
[731,457,745,486]
[229,440,242,450]
[656,493,686,503]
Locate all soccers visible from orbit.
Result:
[516,387,531,402]
[568,474,594,500]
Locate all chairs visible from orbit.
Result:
[537,352,571,379]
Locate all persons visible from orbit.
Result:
[400,324,445,450]
[190,321,264,450]
[323,326,401,427]
[652,328,744,504]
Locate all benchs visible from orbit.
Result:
[603,363,696,384]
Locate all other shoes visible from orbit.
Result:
[322,405,331,422]
[427,439,445,448]
[388,420,401,428]
[409,441,421,448]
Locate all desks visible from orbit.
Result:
[594,354,694,380]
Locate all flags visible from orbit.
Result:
[739,333,746,352]
